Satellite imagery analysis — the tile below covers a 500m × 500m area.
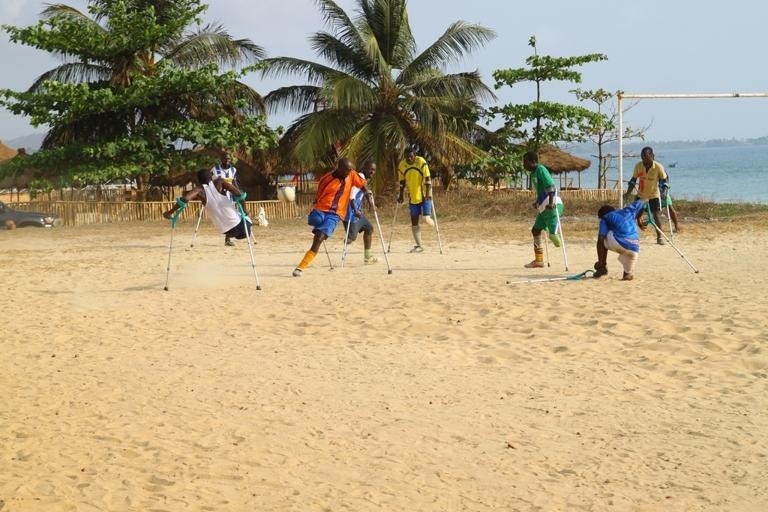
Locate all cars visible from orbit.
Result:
[0,201,61,229]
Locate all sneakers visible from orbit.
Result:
[657,237,665,245]
[256,206,268,227]
[524,261,544,268]
[364,256,377,264]
[410,245,423,253]
[593,267,608,277]
[293,269,302,276]
[226,241,235,246]
[622,273,634,280]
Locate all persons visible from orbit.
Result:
[292,155,378,276]
[396,145,435,253]
[341,160,382,265]
[161,167,270,240]
[623,146,668,245]
[653,153,679,233]
[592,197,651,280]
[207,152,239,247]
[522,152,565,268]
